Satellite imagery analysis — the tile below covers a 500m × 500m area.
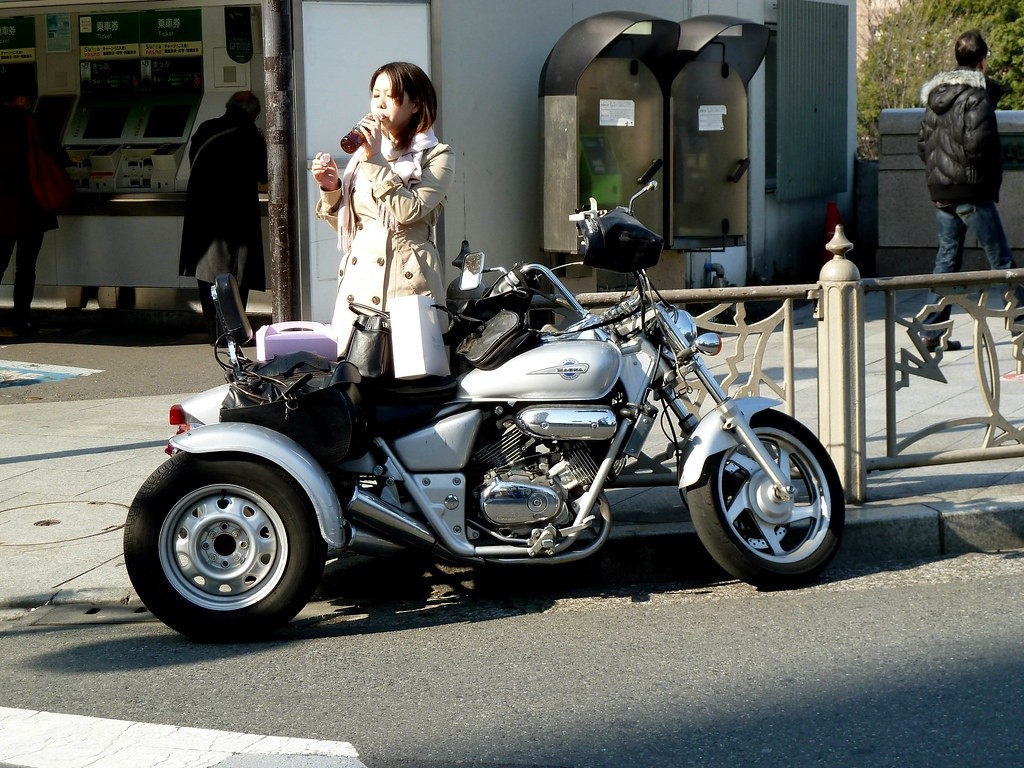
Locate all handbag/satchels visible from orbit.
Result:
[26,144,73,216]
[338,300,391,378]
[456,308,541,371]
[390,295,455,380]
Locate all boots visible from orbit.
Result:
[12,269,36,335]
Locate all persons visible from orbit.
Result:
[177,92,268,347]
[0,74,70,330]
[309,63,454,356]
[917,32,1023,351]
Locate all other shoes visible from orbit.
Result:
[208,316,216,338]
[919,337,962,353]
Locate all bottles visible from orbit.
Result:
[341,113,382,154]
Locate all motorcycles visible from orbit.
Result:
[123,180,845,646]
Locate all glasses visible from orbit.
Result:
[256,115,260,121]
